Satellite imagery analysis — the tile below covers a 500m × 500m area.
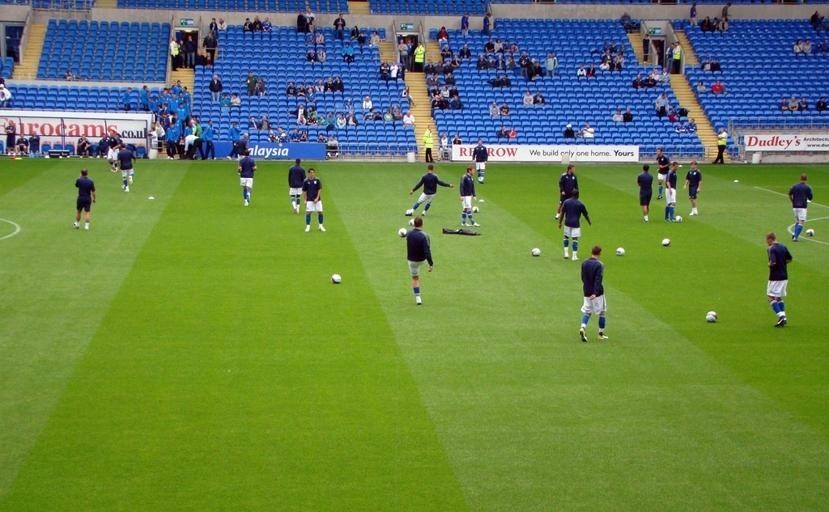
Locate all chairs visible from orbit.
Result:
[0,1,829,162]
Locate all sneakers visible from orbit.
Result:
[579,328,587,341]
[463,222,479,227]
[774,317,786,328]
[664,219,677,223]
[599,333,608,340]
[572,256,579,261]
[304,224,311,232]
[690,210,698,216]
[319,225,326,232]
[416,297,421,305]
[422,211,426,216]
[565,254,568,258]
[245,194,251,206]
[293,206,300,215]
[125,188,130,192]
[791,234,797,242]
[128,175,133,182]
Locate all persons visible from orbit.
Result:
[767,231,792,327]
[781,95,829,111]
[423,123,594,182]
[168,17,272,69]
[712,128,727,164]
[688,2,733,33]
[794,10,829,53]
[559,189,592,262]
[209,61,416,159]
[555,164,578,220]
[297,6,380,63]
[406,165,480,227]
[575,10,697,133]
[238,150,327,233]
[406,217,434,305]
[399,11,557,118]
[0,66,215,231]
[789,173,813,241]
[637,148,702,223]
[697,57,724,94]
[579,246,609,342]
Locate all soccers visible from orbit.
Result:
[661,239,669,246]
[409,219,415,225]
[616,248,625,255]
[532,248,540,255]
[706,310,718,321]
[674,215,682,223]
[73,222,77,227]
[472,206,479,212]
[399,229,407,236]
[332,273,341,283]
[804,228,814,237]
